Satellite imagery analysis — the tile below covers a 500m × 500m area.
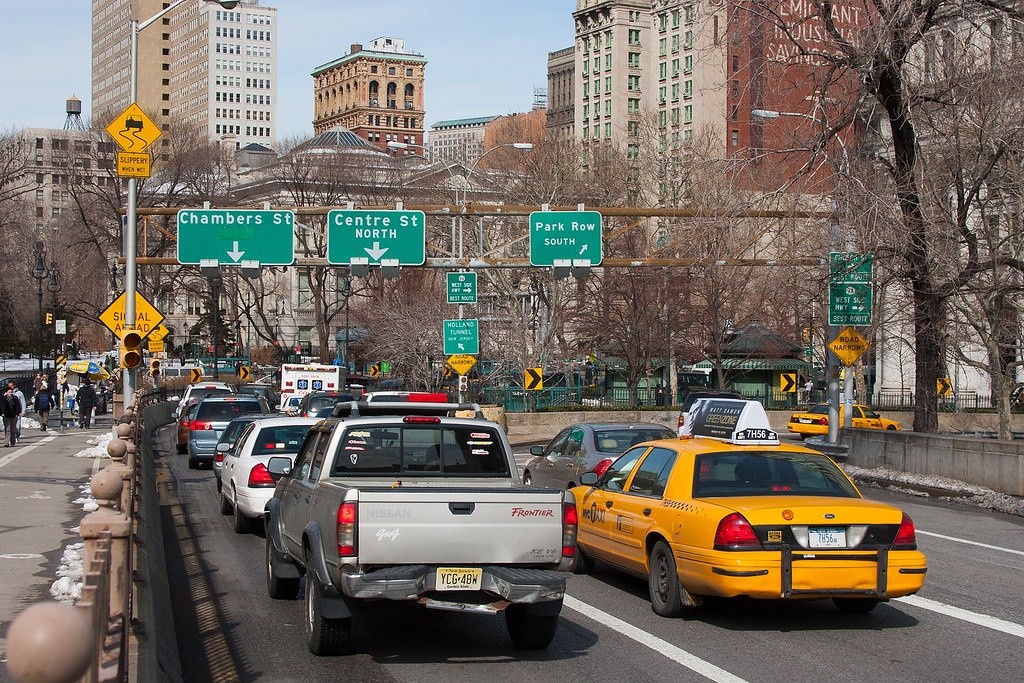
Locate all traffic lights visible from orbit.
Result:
[120,329,143,370]
[46,312,53,325]
[149,358,159,377]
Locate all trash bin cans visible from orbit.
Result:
[939,402,955,412]
[66,396,75,408]
[290,355,295,363]
[381,359,392,373]
[655,389,664,406]
[94,394,107,415]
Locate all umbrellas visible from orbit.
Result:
[69,360,111,381]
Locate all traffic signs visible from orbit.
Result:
[827,282,874,327]
[447,272,477,303]
[177,209,295,266]
[529,210,602,268]
[326,209,425,267]
[443,319,479,354]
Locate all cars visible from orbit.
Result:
[162,356,447,535]
[995,386,1024,414]
[522,421,678,491]
[570,398,928,617]
[787,393,903,441]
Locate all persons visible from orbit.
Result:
[34,385,54,431]
[97,355,115,374]
[76,378,96,429]
[251,362,259,377]
[804,379,813,401]
[0,380,27,447]
[677,379,689,402]
[272,367,282,386]
[655,383,664,406]
[235,360,242,376]
[33,363,60,410]
[179,352,185,366]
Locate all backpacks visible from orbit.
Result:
[38,391,50,408]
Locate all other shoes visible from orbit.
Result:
[4,443,10,447]
[11,443,15,447]
[86,424,89,429]
[15,437,19,444]
[80,422,84,429]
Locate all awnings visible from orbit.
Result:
[696,357,809,370]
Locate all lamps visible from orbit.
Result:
[350,257,370,277]
[241,260,262,279]
[199,259,220,278]
[552,259,572,277]
[572,259,591,277]
[380,258,399,278]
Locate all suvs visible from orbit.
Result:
[677,391,744,438]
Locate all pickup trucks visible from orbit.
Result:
[263,402,579,657]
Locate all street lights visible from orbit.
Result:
[46,258,63,367]
[387,142,534,405]
[31,242,50,374]
[341,275,354,368]
[751,108,855,430]
[527,276,540,344]
[122,0,242,414]
[183,321,189,349]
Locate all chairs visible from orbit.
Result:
[630,435,647,445]
[208,406,227,417]
[733,455,772,483]
[426,444,467,472]
[357,449,400,473]
[257,430,281,443]
[599,438,618,449]
